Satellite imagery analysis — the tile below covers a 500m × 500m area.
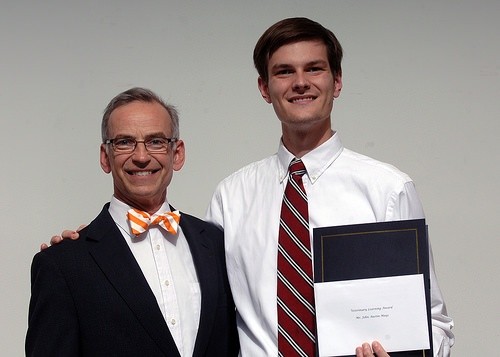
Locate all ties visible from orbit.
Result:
[274,160,319,357]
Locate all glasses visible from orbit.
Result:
[103,135,178,151]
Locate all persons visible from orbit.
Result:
[24,87,240,356]
[40,16,453,356]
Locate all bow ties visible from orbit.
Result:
[127,208,182,235]
